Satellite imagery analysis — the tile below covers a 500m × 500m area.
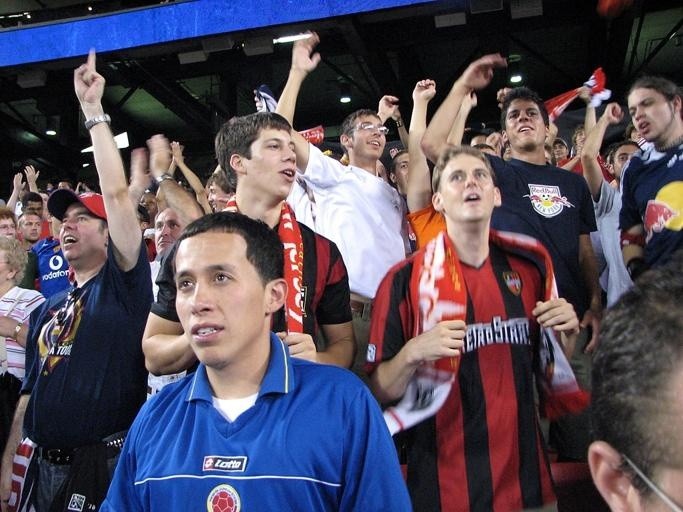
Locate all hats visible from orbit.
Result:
[554,138,567,149]
[47,188,107,221]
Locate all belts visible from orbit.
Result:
[42,429,129,464]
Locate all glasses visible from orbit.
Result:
[357,122,388,134]
[623,453,683,512]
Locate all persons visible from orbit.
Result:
[0,29,683,512]
